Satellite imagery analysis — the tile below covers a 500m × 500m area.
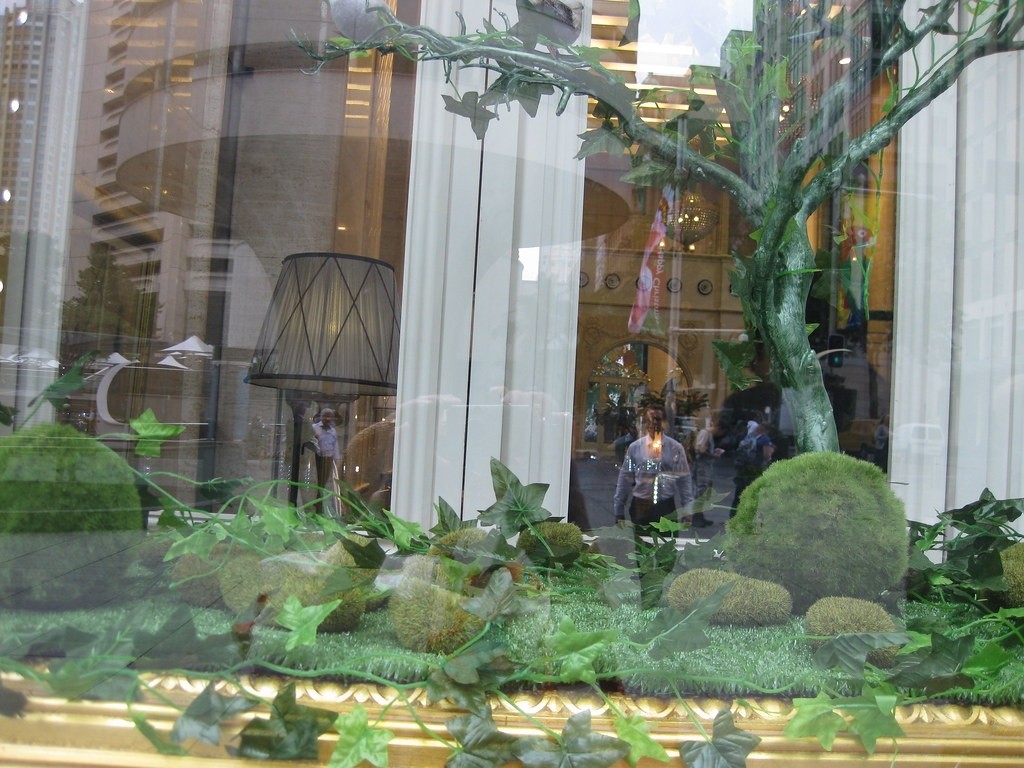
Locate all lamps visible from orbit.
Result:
[243,254,400,514]
[656,132,720,252]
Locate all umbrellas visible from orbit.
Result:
[18,334,215,371]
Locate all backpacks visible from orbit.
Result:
[733,433,767,471]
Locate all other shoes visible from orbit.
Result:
[692,519,713,527]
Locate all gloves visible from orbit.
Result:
[683,429,711,463]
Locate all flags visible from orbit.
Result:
[628,183,675,336]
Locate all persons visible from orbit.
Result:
[609,405,775,610]
[312,407,339,516]
[872,414,890,468]
